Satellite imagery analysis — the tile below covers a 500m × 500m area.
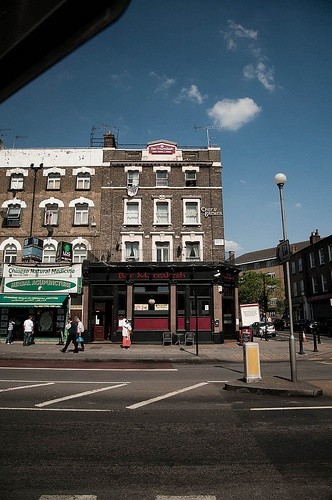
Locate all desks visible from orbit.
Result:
[174,334,184,345]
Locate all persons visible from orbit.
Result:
[123,318,132,351]
[30,315,39,344]
[59,317,78,353]
[23,315,34,346]
[6,317,15,344]
[73,317,85,351]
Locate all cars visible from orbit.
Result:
[294,320,321,334]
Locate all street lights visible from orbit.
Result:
[275,171,297,383]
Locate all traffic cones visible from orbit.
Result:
[302,331,308,342]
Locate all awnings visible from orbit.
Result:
[0,294,69,308]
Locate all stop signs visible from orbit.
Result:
[275,240,292,266]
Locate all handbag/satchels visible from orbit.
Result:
[76,336,84,343]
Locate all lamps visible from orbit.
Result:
[46,224,53,233]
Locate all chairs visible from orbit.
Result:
[184,332,195,346]
[163,332,172,346]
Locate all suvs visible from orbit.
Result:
[273,319,290,331]
[249,321,277,337]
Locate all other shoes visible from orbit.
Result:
[27,343,31,346]
[11,342,13,344]
[23,343,26,346]
[31,341,35,344]
[59,349,65,353]
[6,342,10,344]
